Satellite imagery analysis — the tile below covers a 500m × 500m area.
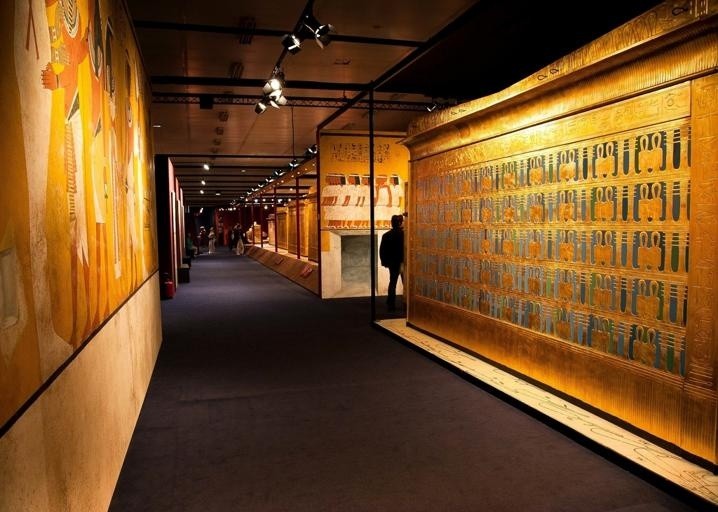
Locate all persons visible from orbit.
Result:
[321,172,405,230]
[262,231,269,242]
[187,233,197,255]
[207,227,216,254]
[235,225,244,256]
[198,226,207,251]
[379,214,404,311]
[227,225,237,252]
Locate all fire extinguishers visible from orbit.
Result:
[162,272,175,299]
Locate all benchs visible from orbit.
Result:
[182,256,191,268]
[179,264,190,283]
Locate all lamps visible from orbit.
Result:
[303,145,317,159]
[216,191,221,196]
[427,103,440,112]
[246,190,252,195]
[288,159,298,171]
[274,169,286,177]
[240,196,245,199]
[258,183,267,188]
[254,12,337,115]
[251,187,259,192]
[265,177,276,183]
